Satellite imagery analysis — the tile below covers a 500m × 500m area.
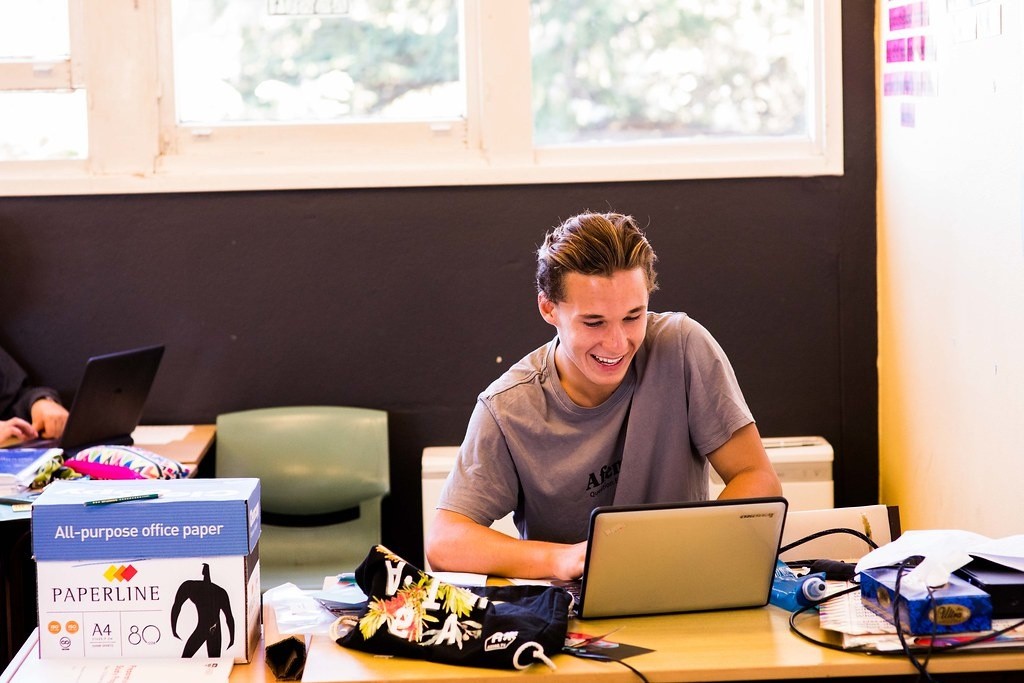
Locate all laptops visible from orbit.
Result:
[546,497,790,620]
[0,344,169,462]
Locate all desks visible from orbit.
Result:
[0,575,1024,683]
[1,423,218,672]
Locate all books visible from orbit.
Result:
[0,449,64,486]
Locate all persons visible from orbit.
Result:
[425,212,783,581]
[0,348,70,444]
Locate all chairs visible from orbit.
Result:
[215,405,390,590]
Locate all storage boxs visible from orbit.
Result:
[412,444,521,574]
[702,435,837,521]
[31,478,262,663]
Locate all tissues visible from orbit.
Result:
[859,535,992,637]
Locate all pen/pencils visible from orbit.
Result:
[83,493,164,507]
